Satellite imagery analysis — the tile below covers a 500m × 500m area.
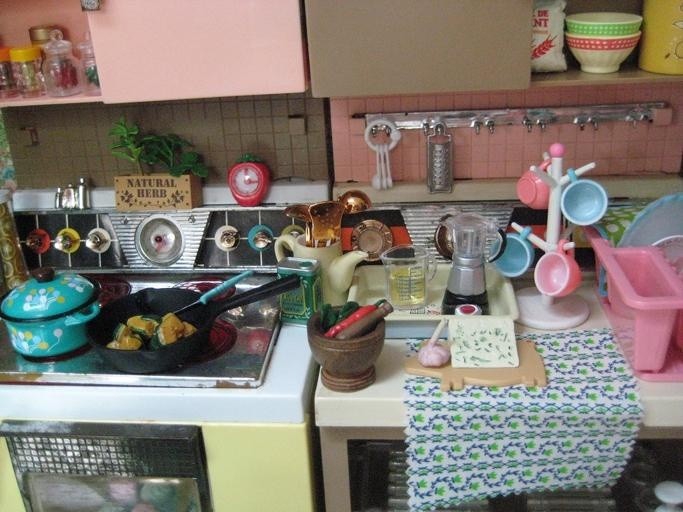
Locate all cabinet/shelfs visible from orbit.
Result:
[0,0,309,110]
[302,0,682,99]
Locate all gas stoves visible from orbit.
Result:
[0,271,309,395]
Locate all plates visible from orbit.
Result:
[653,232,681,268]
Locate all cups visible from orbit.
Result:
[489,222,537,279]
[381,241,438,311]
[531,239,581,301]
[560,168,610,227]
[515,157,552,212]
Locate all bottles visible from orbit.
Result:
[0,23,103,99]
[54,178,95,210]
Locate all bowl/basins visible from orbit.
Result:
[0,268,102,362]
[562,10,644,76]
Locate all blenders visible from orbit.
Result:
[441,216,507,317]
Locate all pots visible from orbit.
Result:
[86,272,301,375]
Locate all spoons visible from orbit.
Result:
[372,141,394,194]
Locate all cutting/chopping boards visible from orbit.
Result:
[406,333,547,395]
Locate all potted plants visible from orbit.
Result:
[107,112,208,211]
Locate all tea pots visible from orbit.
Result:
[274,231,367,306]
